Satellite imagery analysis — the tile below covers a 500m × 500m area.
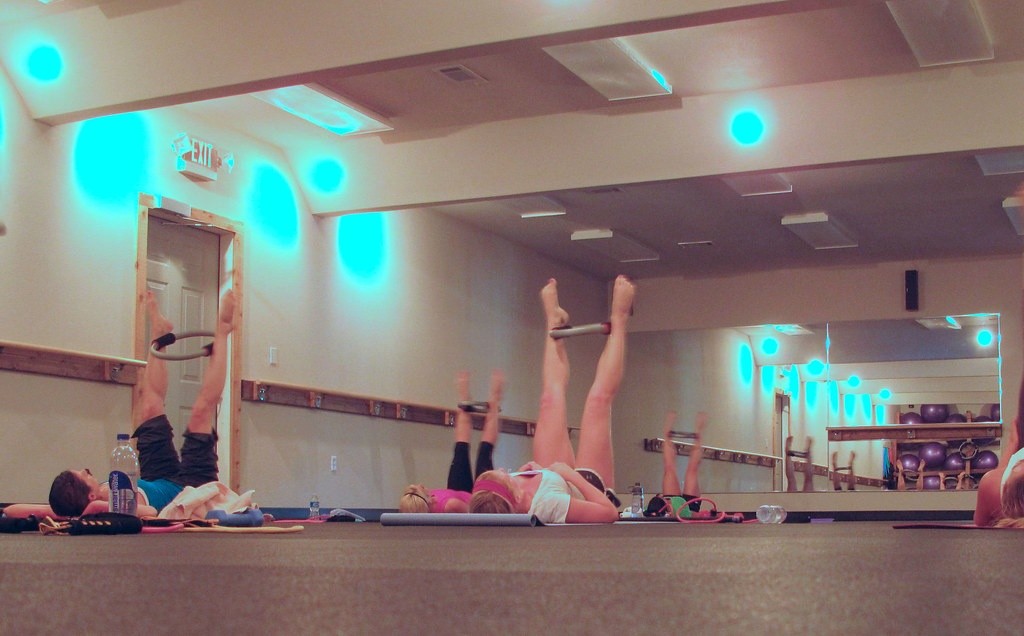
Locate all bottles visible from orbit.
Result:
[623,507,632,512]
[108,433,138,517]
[309,493,320,516]
[620,512,631,518]
[756,505,787,523]
[632,482,644,514]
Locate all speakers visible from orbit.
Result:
[905,270,919,312]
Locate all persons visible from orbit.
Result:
[400,366,506,513]
[785,435,814,491]
[973,375,1024,528]
[469,275,634,523]
[3,290,236,520]
[643,410,719,520]
[831,451,856,490]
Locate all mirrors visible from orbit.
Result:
[611,314,1002,495]
